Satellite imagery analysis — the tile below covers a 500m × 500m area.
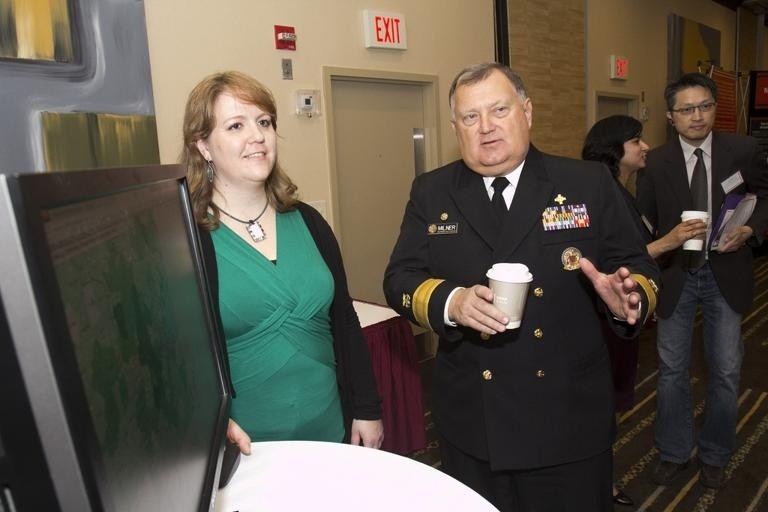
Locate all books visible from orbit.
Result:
[706,193,756,251]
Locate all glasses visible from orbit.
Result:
[672,102,715,115]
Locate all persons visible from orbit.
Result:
[581,115,705,503]
[185,73,388,455]
[382,64,660,512]
[635,74,767,491]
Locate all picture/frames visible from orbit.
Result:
[667,10,722,141]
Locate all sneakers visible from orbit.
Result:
[699,463,724,488]
[649,461,680,484]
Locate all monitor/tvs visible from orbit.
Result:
[0,164,241,512]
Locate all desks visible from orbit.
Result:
[207,439,502,512]
[353,298,428,455]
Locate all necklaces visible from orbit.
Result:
[204,191,274,243]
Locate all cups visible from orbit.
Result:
[680,210,709,251]
[487,261,534,330]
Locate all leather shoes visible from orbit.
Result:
[613,490,633,506]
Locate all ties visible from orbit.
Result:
[689,148,708,274]
[491,177,510,213]
